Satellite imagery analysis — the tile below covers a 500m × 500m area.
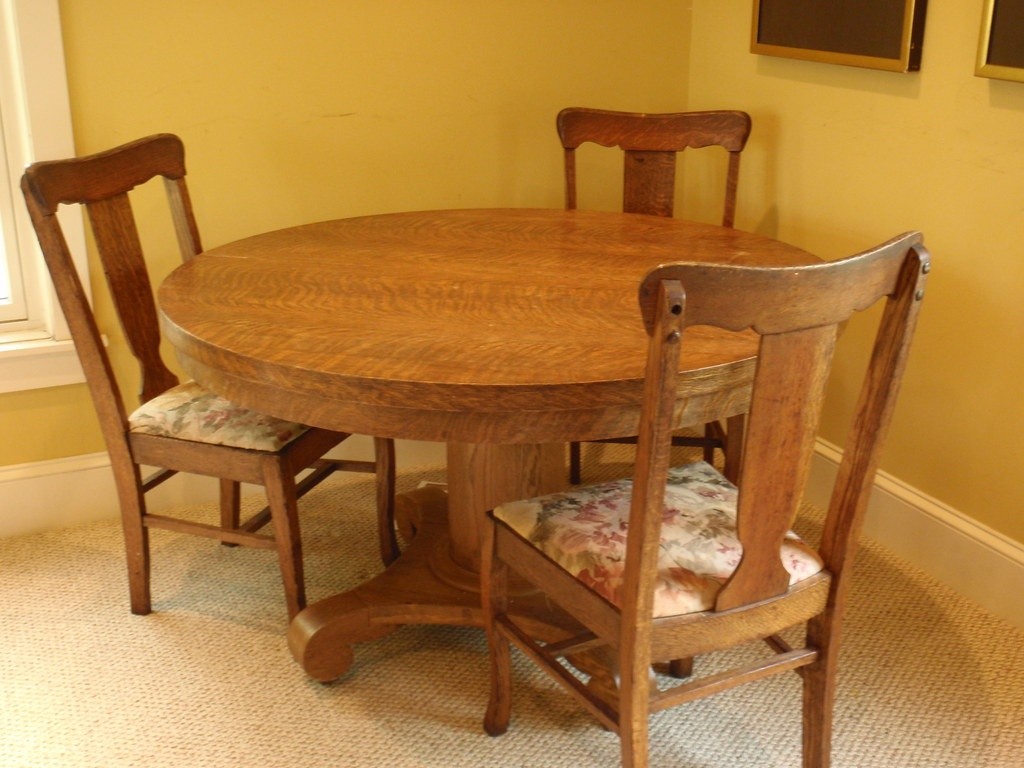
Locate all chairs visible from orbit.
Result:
[484,231,930,767]
[18,132,403,624]
[555,106,752,484]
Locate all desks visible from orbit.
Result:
[155,209,846,733]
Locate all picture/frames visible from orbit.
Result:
[749,0,927,74]
[974,0,1024,82]
